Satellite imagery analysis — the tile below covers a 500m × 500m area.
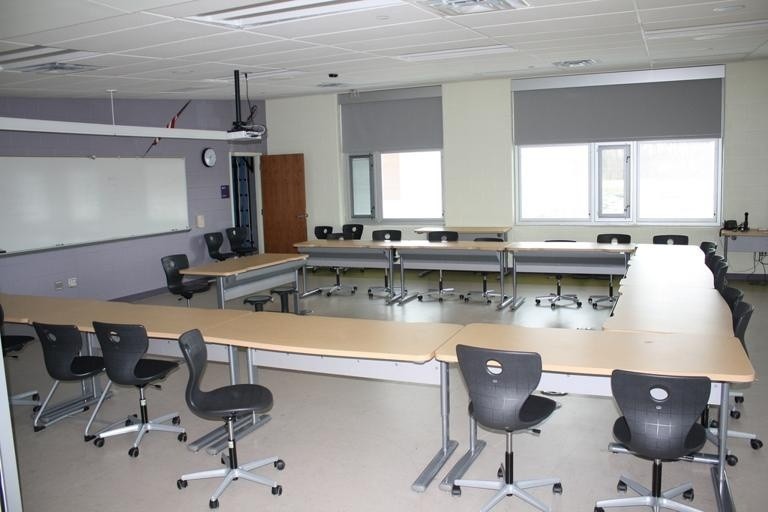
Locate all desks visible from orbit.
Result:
[29,302,252,451]
[435,323,755,512]
[1,294,107,421]
[202,311,464,492]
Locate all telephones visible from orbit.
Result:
[724,220,737,230]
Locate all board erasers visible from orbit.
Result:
[0,250,6,253]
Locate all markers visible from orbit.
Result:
[56,244,64,246]
[172,229,179,231]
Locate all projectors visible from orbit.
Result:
[227,131,262,142]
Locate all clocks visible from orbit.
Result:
[203,147,217,167]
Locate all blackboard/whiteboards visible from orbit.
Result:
[0,155,191,258]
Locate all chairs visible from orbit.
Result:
[1,306,42,415]
[450,344,563,512]
[594,368,713,512]
[159,223,754,357]
[31,321,138,442]
[701,300,764,466]
[177,329,285,509]
[92,321,187,458]
